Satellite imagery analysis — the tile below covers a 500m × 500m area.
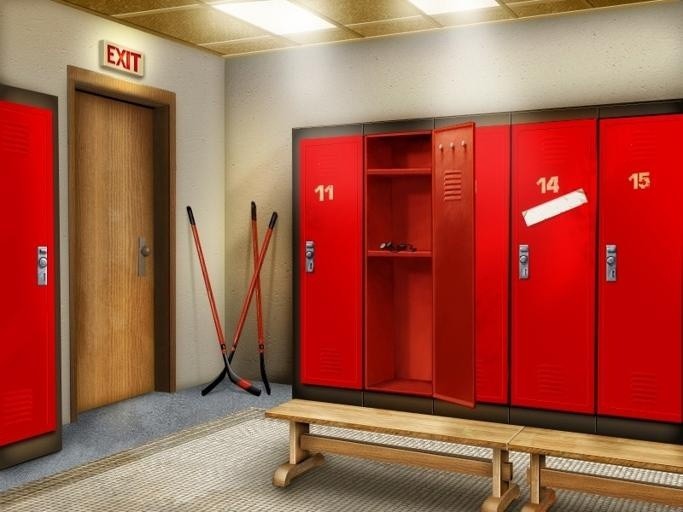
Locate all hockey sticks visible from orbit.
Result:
[187,201,277,396]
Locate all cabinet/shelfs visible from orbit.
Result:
[0,84,63,469]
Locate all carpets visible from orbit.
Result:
[0,404,681,512]
